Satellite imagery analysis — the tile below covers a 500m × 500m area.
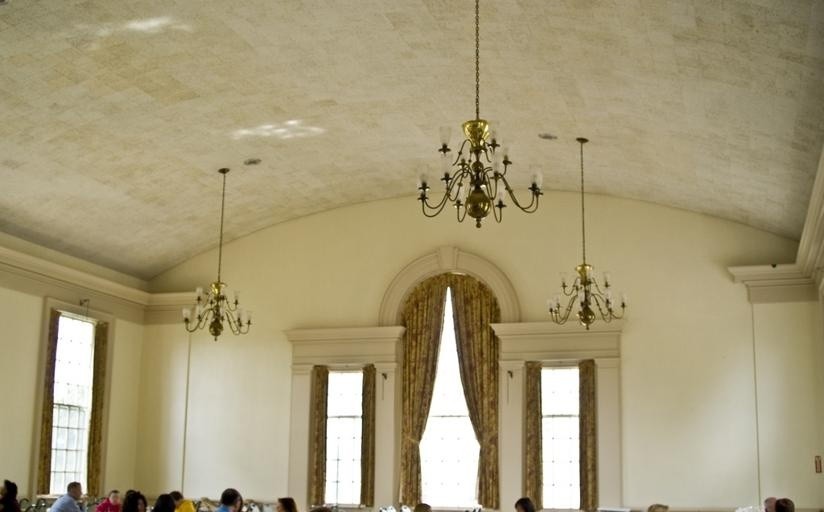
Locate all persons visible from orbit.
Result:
[0,479,23,511]
[95,478,329,512]
[48,482,87,511]
[413,502,434,511]
[774,498,796,511]
[513,497,534,512]
[764,496,778,511]
[645,504,668,512]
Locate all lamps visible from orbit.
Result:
[180,165,257,345]
[546,135,627,334]
[411,0,547,228]
